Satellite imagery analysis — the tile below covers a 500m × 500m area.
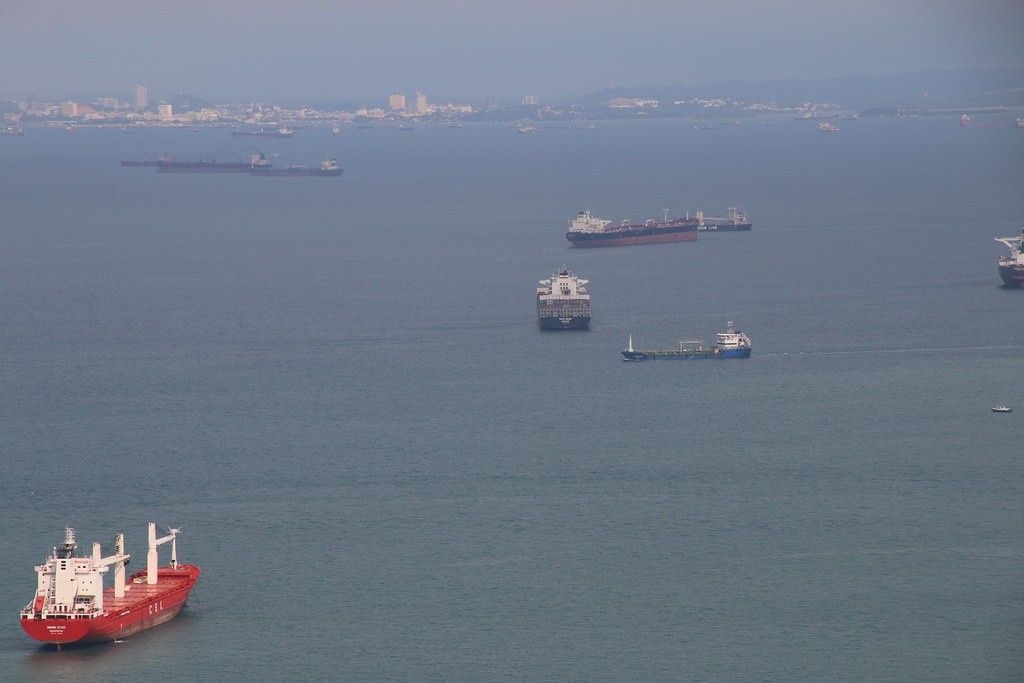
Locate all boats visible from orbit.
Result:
[230,125,296,138]
[566,209,699,248]
[992,405,1012,413]
[537,264,591,330]
[696,207,752,232]
[120,152,252,173]
[250,152,345,177]
[333,124,340,135]
[18,522,201,650]
[506,118,536,133]
[398,127,417,130]
[620,320,752,361]
[817,122,840,131]
[448,121,462,128]
[994,228,1024,289]
